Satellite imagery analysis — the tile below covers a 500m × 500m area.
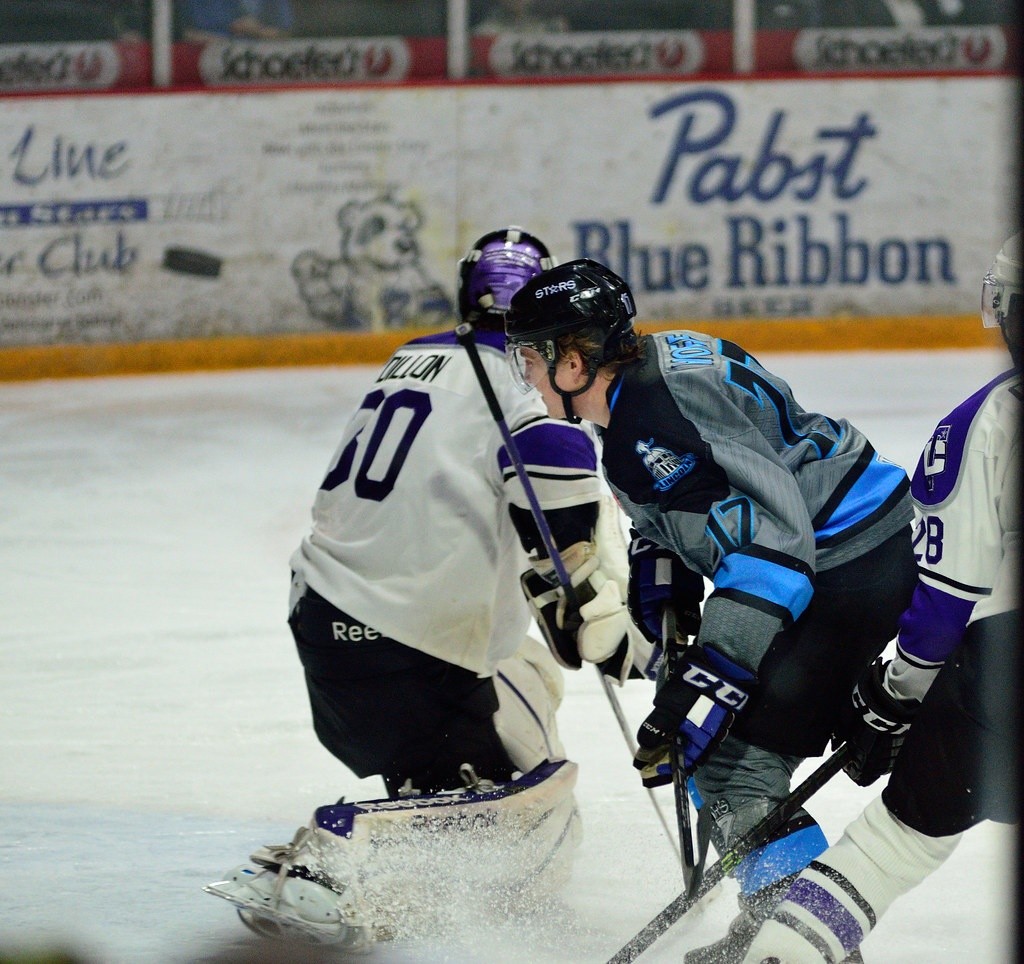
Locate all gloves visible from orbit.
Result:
[832,658,917,787]
[627,522,705,650]
[633,643,755,788]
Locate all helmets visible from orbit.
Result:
[456,226,556,325]
[992,232,1024,293]
[504,258,637,345]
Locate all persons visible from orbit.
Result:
[738,231,1024,963]
[115,0,994,64]
[506,258,919,963]
[206,225,677,953]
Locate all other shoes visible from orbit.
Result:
[685,892,863,964]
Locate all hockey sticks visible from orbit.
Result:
[603,740,861,964]
[458,319,713,903]
